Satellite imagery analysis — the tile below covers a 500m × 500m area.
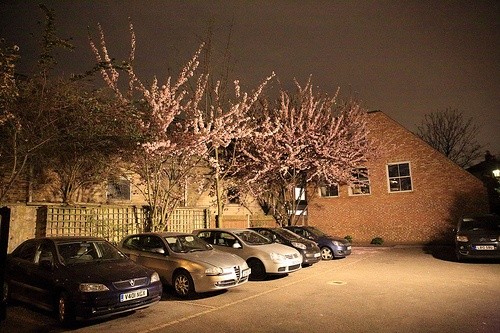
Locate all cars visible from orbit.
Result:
[113,233,252,298]
[0,236,164,329]
[284,225,352,261]
[452,214,500,263]
[247,226,322,268]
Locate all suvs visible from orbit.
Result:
[191,229,305,279]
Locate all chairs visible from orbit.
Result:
[40,250,65,264]
[169,243,183,252]
[71,246,95,262]
